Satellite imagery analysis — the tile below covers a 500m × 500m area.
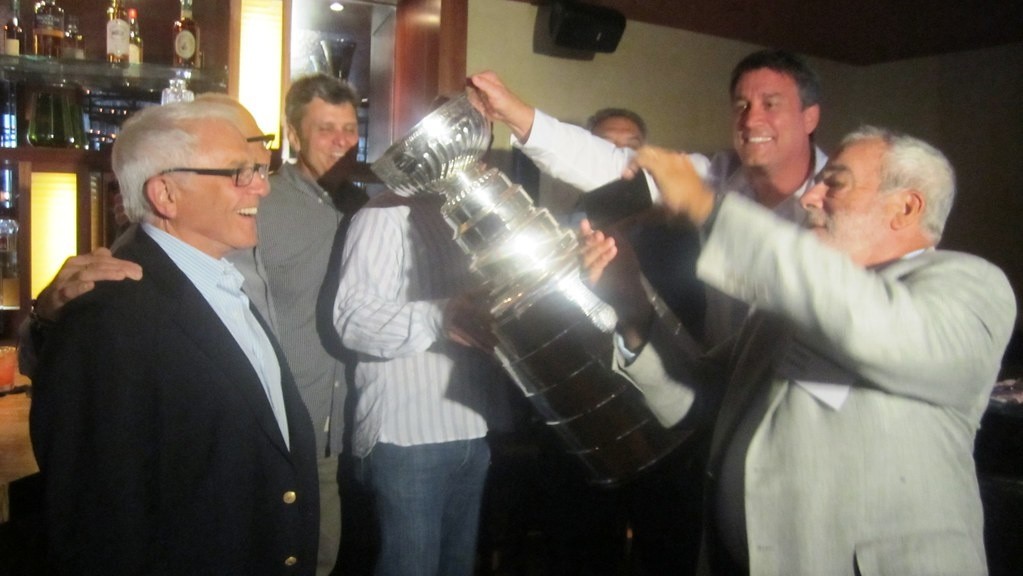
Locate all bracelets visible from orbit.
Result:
[27,298,39,321]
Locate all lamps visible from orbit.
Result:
[16,160,109,323]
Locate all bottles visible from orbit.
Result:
[0,192,19,278]
[106,0,143,87]
[4,1,26,69]
[173,0,202,78]
[32,1,85,74]
[92,97,151,142]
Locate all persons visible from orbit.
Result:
[578,127,1017,576]
[585,107,648,151]
[258,72,389,576]
[31,102,323,576]
[332,190,491,576]
[464,49,832,353]
[20,91,278,381]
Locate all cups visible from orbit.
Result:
[26,79,86,148]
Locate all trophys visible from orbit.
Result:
[373,96,722,484]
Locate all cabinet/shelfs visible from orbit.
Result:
[0,0,240,347]
